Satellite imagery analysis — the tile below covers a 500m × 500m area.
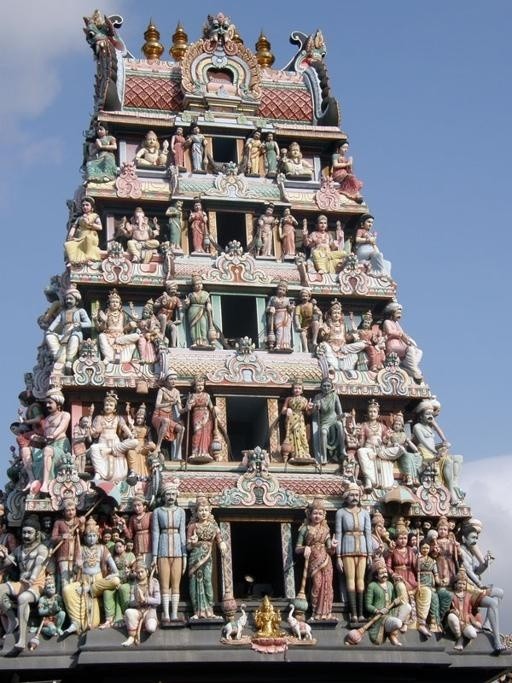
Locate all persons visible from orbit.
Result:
[1,483,507,652]
[10,284,451,490]
[64,122,393,280]
